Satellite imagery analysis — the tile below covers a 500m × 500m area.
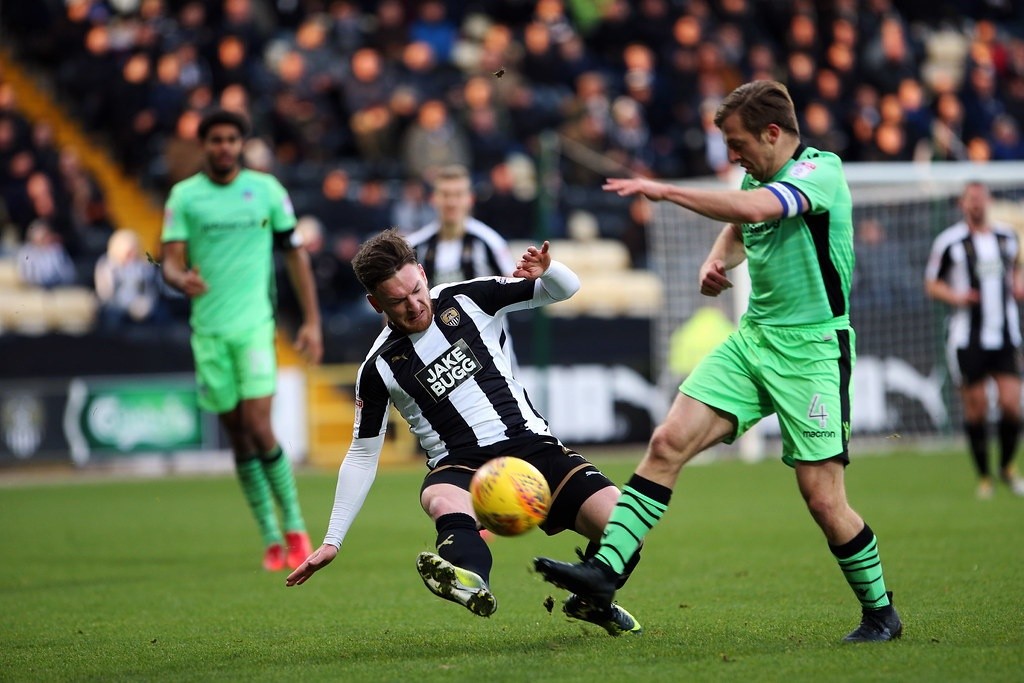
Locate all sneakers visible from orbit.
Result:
[561,594,643,637]
[531,557,618,608]
[415,552,496,618]
[844,606,902,644]
[263,533,312,570]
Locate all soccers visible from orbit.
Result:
[468,454,553,538]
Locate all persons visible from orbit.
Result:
[528,81,902,647]
[922,180,1023,501]
[0,0,1024,331]
[285,228,643,638]
[401,164,520,380]
[160,109,322,573]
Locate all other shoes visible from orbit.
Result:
[972,477,1023,497]
